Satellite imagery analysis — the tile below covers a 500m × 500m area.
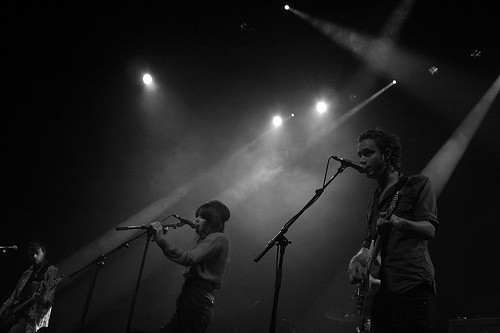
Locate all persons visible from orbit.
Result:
[150,200,230,333]
[348,129,438,333]
[0,240,59,332]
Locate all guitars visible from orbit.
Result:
[353,190,404,333]
[0,288,45,333]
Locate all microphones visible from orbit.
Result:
[172,214,198,228]
[331,155,367,173]
[0,245,18,250]
